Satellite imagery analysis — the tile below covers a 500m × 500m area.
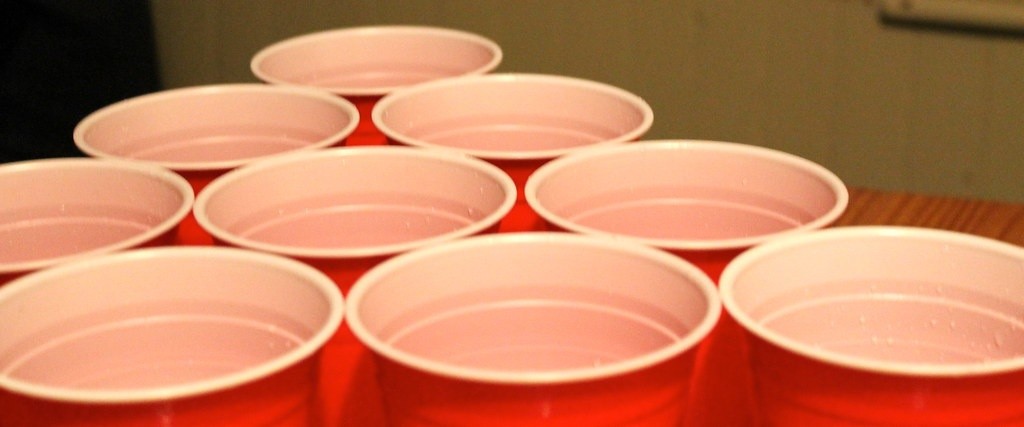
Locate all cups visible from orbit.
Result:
[0,157,195,292]
[525,139,850,427]
[0,245,344,427]
[193,147,518,427]
[343,233,719,427]
[371,73,655,233]
[719,225,1024,427]
[73,83,360,245]
[250,27,503,148]
